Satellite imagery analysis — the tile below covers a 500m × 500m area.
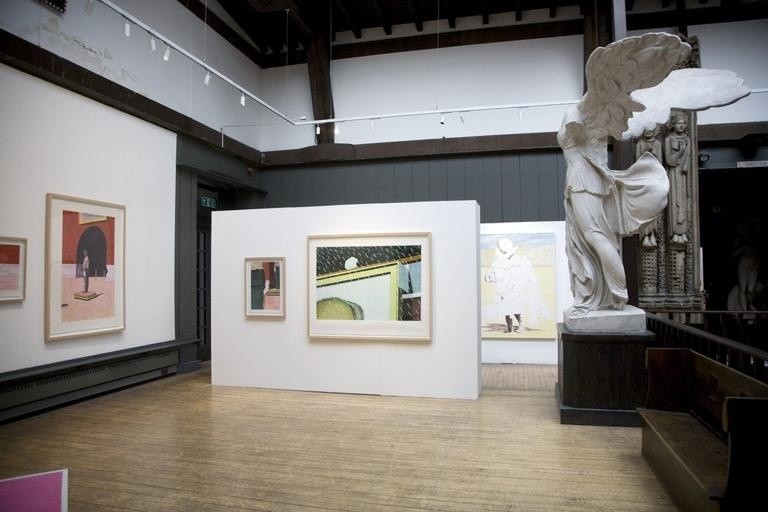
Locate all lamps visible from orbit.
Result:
[123,19,172,62]
[315,124,322,136]
[85,1,96,16]
[203,71,211,86]
[239,93,246,107]
[440,115,446,125]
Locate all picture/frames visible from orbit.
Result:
[244,257,286,319]
[43,192,127,341]
[306,232,433,344]
[0,236,28,303]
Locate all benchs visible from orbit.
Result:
[635,346,767,512]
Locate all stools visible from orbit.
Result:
[0,467,69,511]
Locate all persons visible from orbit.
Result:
[635,124,664,250]
[731,221,758,313]
[664,110,692,249]
[486,239,530,333]
[251,262,271,308]
[556,105,630,312]
[81,248,90,294]
